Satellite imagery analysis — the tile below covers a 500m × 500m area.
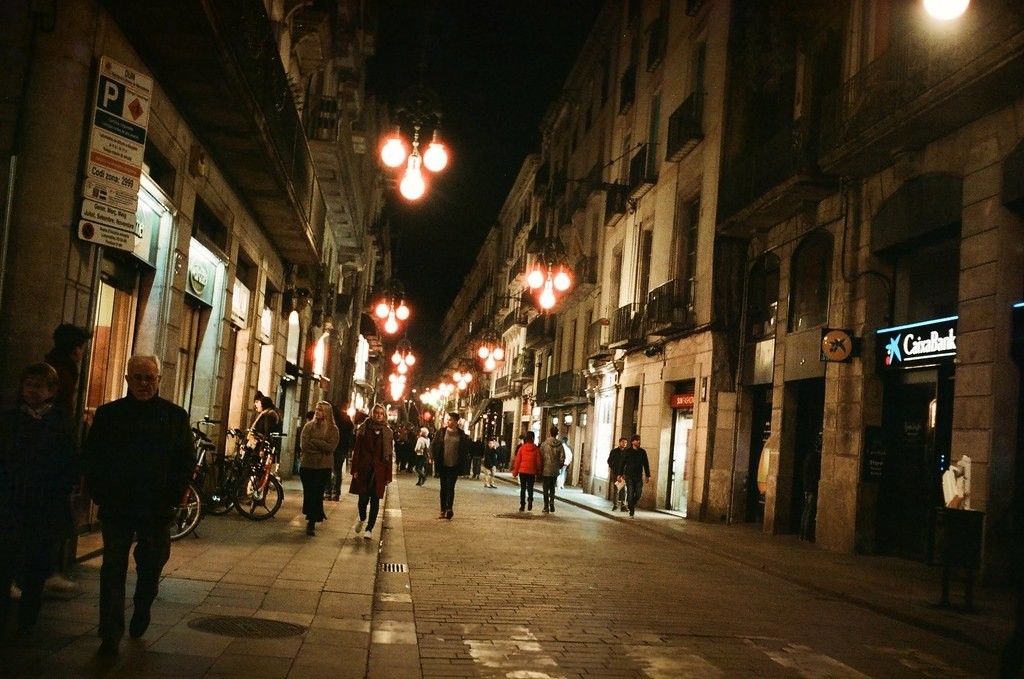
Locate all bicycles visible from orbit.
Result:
[168,414,285,541]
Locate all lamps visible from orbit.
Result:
[372,98,619,407]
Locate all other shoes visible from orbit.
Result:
[446,509,453,519]
[438,511,446,518]
[96,638,120,658]
[612,505,618,511]
[542,508,549,512]
[17,615,32,637]
[528,500,532,510]
[550,503,554,512]
[416,482,421,486]
[332,493,339,501]
[323,493,332,500]
[130,596,151,638]
[621,506,629,511]
[355,520,364,533]
[422,477,428,484]
[519,504,525,511]
[630,513,633,516]
[307,520,316,536]
[364,530,371,539]
[484,484,497,489]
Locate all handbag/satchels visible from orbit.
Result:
[423,446,430,459]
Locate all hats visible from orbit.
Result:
[53,324,93,349]
[254,391,264,402]
[630,435,640,441]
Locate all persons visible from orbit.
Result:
[429,412,471,519]
[348,404,393,539]
[244,391,281,507]
[0,359,79,635]
[395,427,440,486]
[299,401,340,537]
[79,355,197,646]
[324,400,354,501]
[16,323,84,406]
[512,427,572,513]
[608,434,651,519]
[461,435,509,488]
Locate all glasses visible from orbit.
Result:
[25,379,51,389]
[129,374,159,383]
